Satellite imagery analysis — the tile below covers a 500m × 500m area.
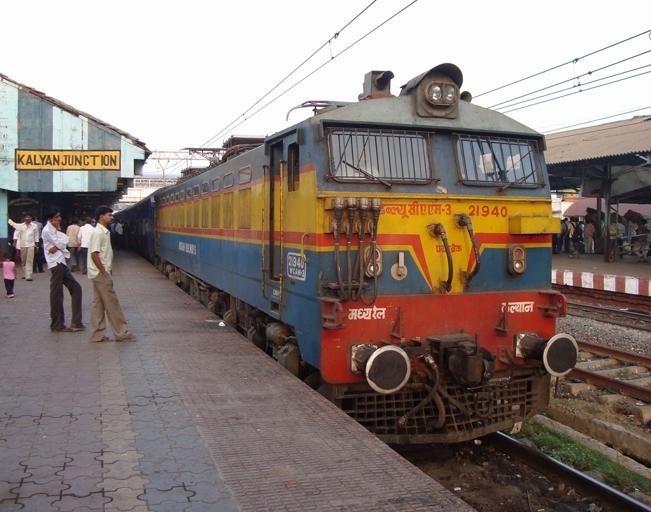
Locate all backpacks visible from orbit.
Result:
[570,225,583,242]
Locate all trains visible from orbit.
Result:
[106,63,579,445]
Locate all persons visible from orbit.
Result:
[0,252,17,298]
[39,205,85,333]
[85,204,139,343]
[7,209,125,281]
[553,208,651,265]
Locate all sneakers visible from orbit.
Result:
[70,322,88,331]
[54,325,74,333]
[71,265,80,271]
[114,334,138,342]
[4,292,15,298]
[92,335,113,342]
[20,275,33,281]
